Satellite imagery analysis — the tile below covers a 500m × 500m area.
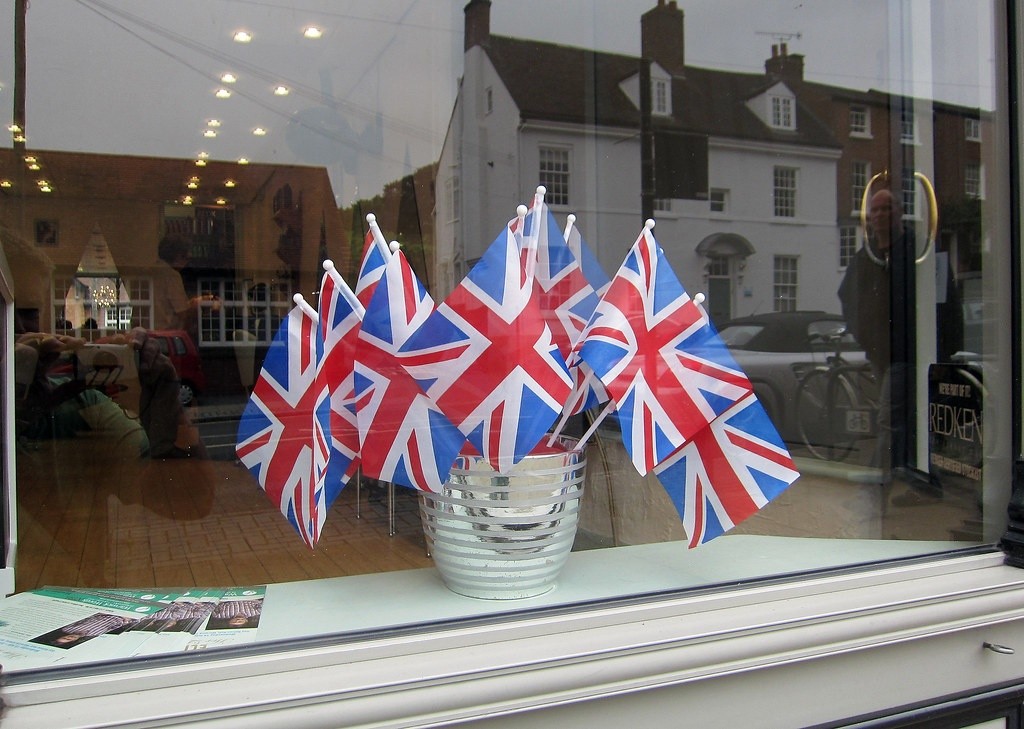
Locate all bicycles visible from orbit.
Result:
[793,323,996,469]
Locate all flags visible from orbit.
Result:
[653,303,801,549]
[354,250,466,494]
[234,306,363,549]
[574,229,751,477]
[313,271,367,544]
[394,197,612,474]
[354,229,386,309]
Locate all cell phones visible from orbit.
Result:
[71,344,139,384]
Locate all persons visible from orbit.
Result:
[158,602,208,628]
[0,230,213,587]
[838,189,965,513]
[56,613,131,643]
[212,600,262,625]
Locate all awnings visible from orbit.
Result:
[695,232,756,257]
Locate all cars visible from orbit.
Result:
[605,309,980,446]
